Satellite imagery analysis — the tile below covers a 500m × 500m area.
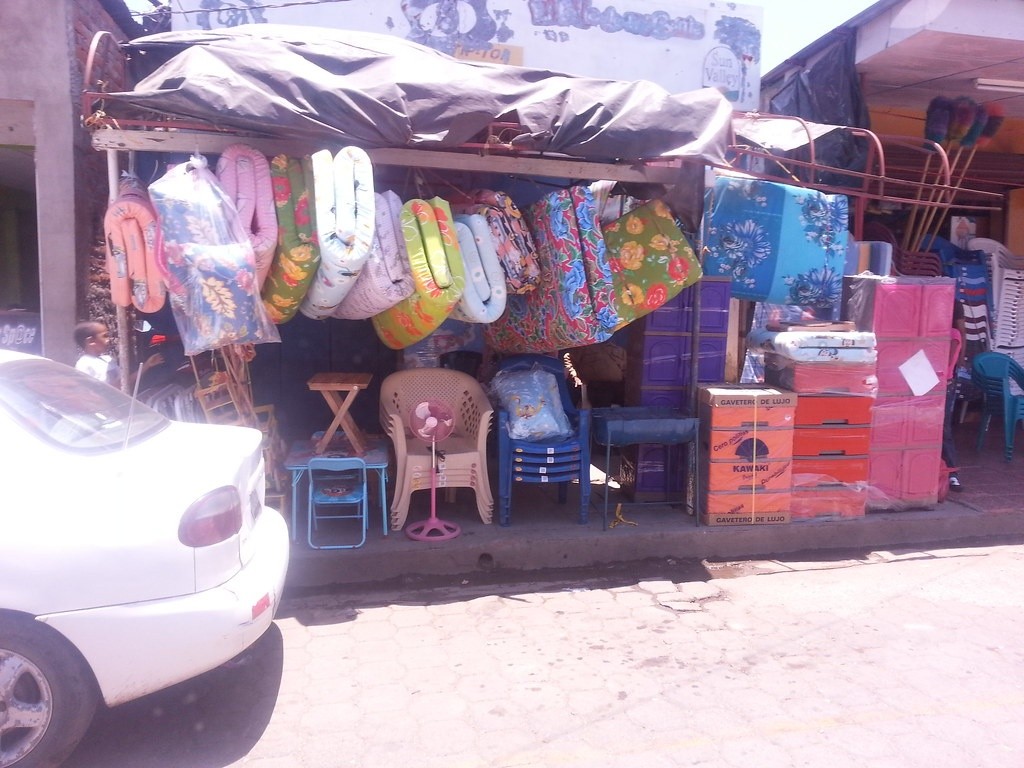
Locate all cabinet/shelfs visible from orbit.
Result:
[841,273,957,509]
[195,374,256,429]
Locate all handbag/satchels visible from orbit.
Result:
[148,154,283,356]
[491,361,576,442]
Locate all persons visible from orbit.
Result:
[73,321,166,388]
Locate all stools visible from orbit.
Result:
[307,373,374,452]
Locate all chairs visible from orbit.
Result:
[379,351,593,531]
[865,220,1024,467]
[304,457,370,550]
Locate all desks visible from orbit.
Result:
[285,437,392,540]
[591,407,700,532]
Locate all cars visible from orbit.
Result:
[1,349,291,768]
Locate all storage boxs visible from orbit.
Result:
[630,270,879,520]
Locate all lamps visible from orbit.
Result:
[972,77,1024,92]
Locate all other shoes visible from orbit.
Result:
[949,476,962,490]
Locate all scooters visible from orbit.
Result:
[131,318,213,383]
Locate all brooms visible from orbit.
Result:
[901,94,1006,270]
